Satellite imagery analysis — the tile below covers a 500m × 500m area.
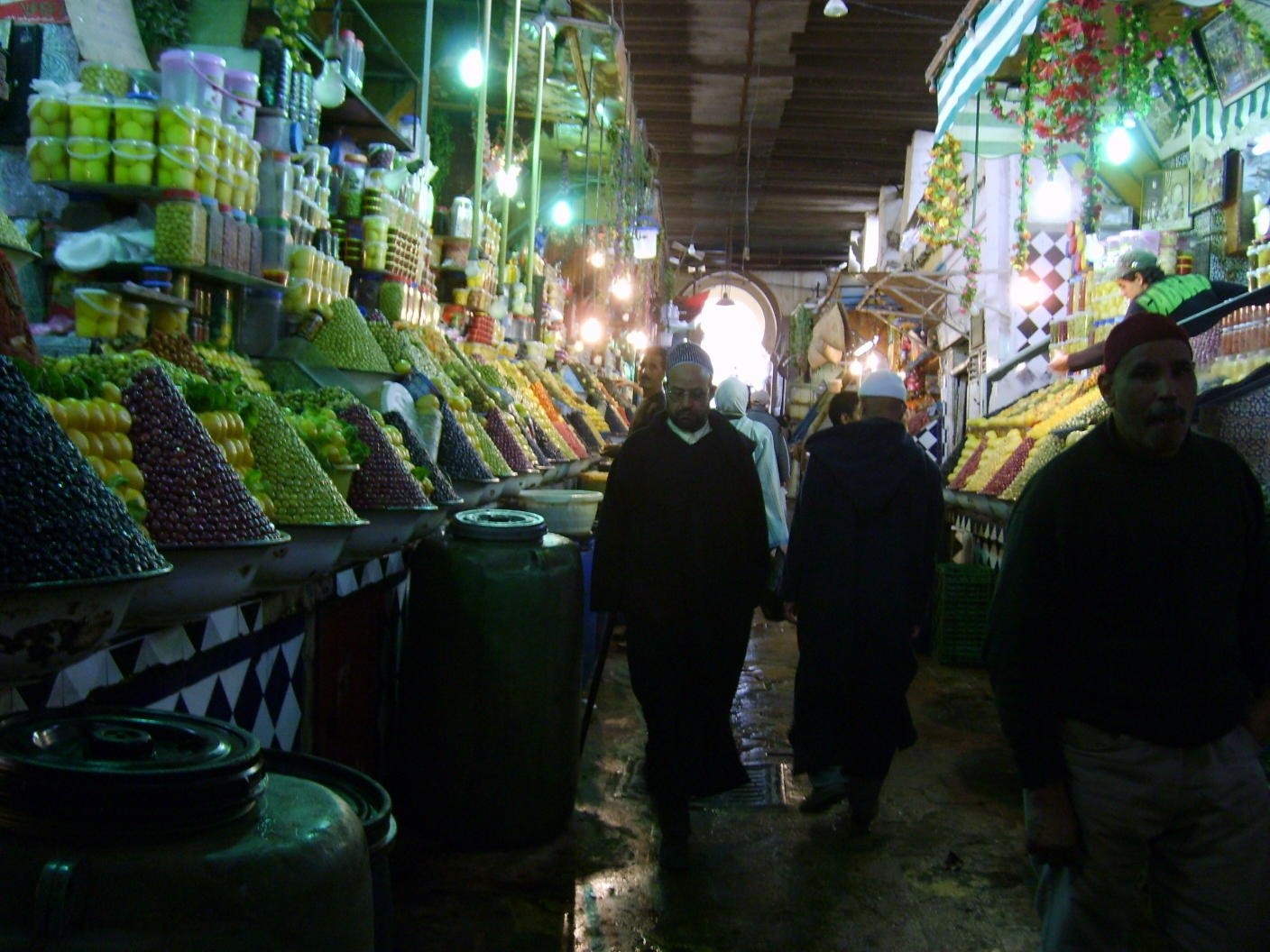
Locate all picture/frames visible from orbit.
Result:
[1188,151,1228,215]
[1139,166,1192,233]
[1199,10,1270,109]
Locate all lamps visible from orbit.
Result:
[1004,81,1024,103]
[823,0,848,18]
[715,228,735,305]
[545,36,567,87]
[521,11,546,38]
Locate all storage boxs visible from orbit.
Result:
[936,563,994,666]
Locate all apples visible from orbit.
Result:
[948,379,1102,490]
[265,0,316,74]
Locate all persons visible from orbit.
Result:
[1047,251,1247,373]
[983,313,1270,952]
[588,343,947,873]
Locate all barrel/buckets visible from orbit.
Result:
[1049,229,1161,379]
[160,49,262,141]
[157,104,263,210]
[0,708,398,952]
[363,217,390,270]
[73,287,123,340]
[237,286,284,357]
[369,141,396,171]
[265,115,353,319]
[150,303,189,338]
[120,300,150,339]
[409,508,601,851]
[26,61,157,190]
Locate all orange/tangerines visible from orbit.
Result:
[280,407,353,464]
[531,380,559,422]
[199,410,275,518]
[449,369,538,475]
[394,358,411,373]
[371,395,438,494]
[34,349,155,537]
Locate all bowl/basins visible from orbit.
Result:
[337,366,399,396]
[432,471,518,532]
[518,489,604,535]
[503,453,603,499]
[0,243,42,272]
[0,560,174,677]
[341,505,438,556]
[159,530,292,621]
[253,519,371,589]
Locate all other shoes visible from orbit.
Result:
[799,785,843,813]
[658,825,693,872]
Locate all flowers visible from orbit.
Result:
[984,0,1270,271]
[915,131,987,313]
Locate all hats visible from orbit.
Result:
[1103,310,1193,374]
[664,342,714,377]
[1120,252,1156,281]
[857,369,907,404]
[750,390,771,406]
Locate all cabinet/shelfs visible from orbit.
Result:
[38,179,289,291]
[433,235,496,278]
[263,0,434,153]
